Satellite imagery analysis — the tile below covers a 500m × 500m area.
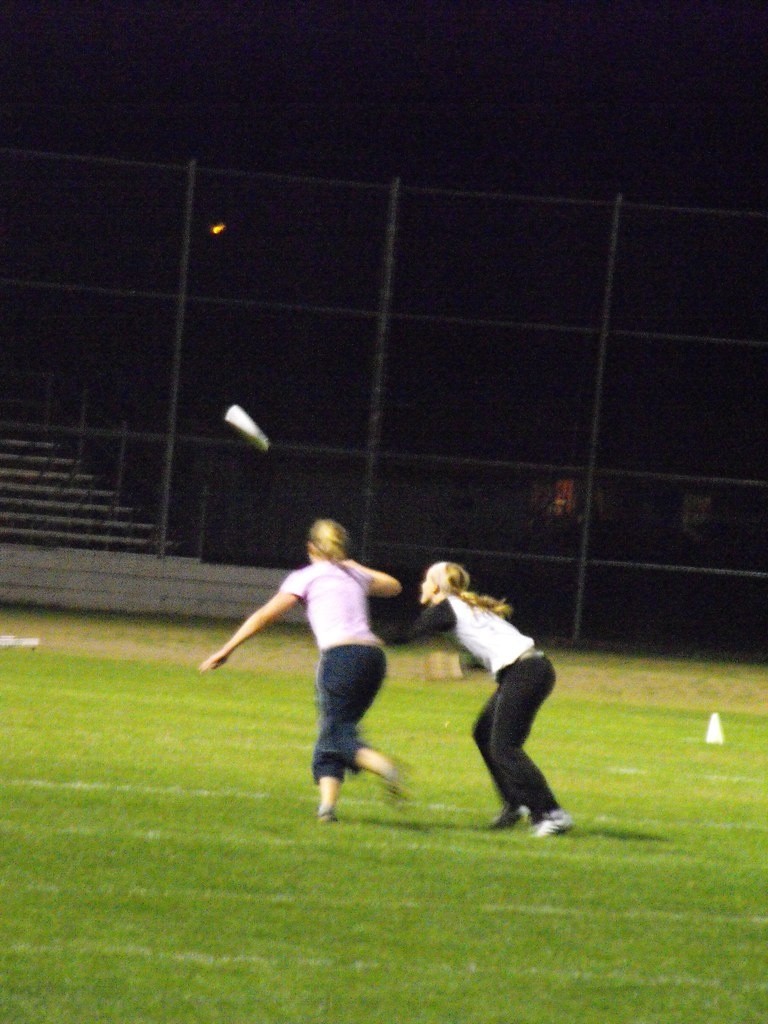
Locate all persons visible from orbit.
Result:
[377,563,575,837]
[199,518,402,823]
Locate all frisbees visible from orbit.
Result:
[223,403,271,452]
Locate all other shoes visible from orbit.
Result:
[532,814,571,837]
[491,806,531,829]
